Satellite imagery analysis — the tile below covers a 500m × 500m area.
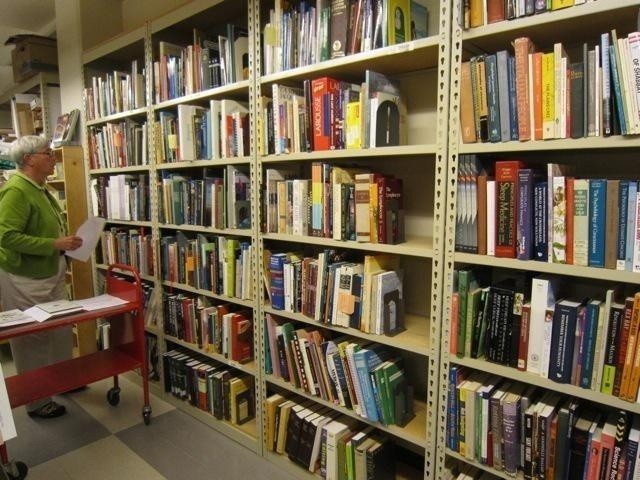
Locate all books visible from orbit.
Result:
[153,23,251,425]
[84,61,159,386]
[263,0,428,480]
[46,163,79,348]
[62,109,80,141]
[446,1,640,480]
[52,114,69,143]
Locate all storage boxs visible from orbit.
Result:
[5,33,59,81]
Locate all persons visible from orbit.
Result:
[0,135,88,418]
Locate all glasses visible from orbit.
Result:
[31,148,52,156]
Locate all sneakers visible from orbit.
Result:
[27,399,65,418]
[0,456,28,480]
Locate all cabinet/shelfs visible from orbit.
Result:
[82,4,638,473]
[1,147,102,368]
[1,73,64,147]
